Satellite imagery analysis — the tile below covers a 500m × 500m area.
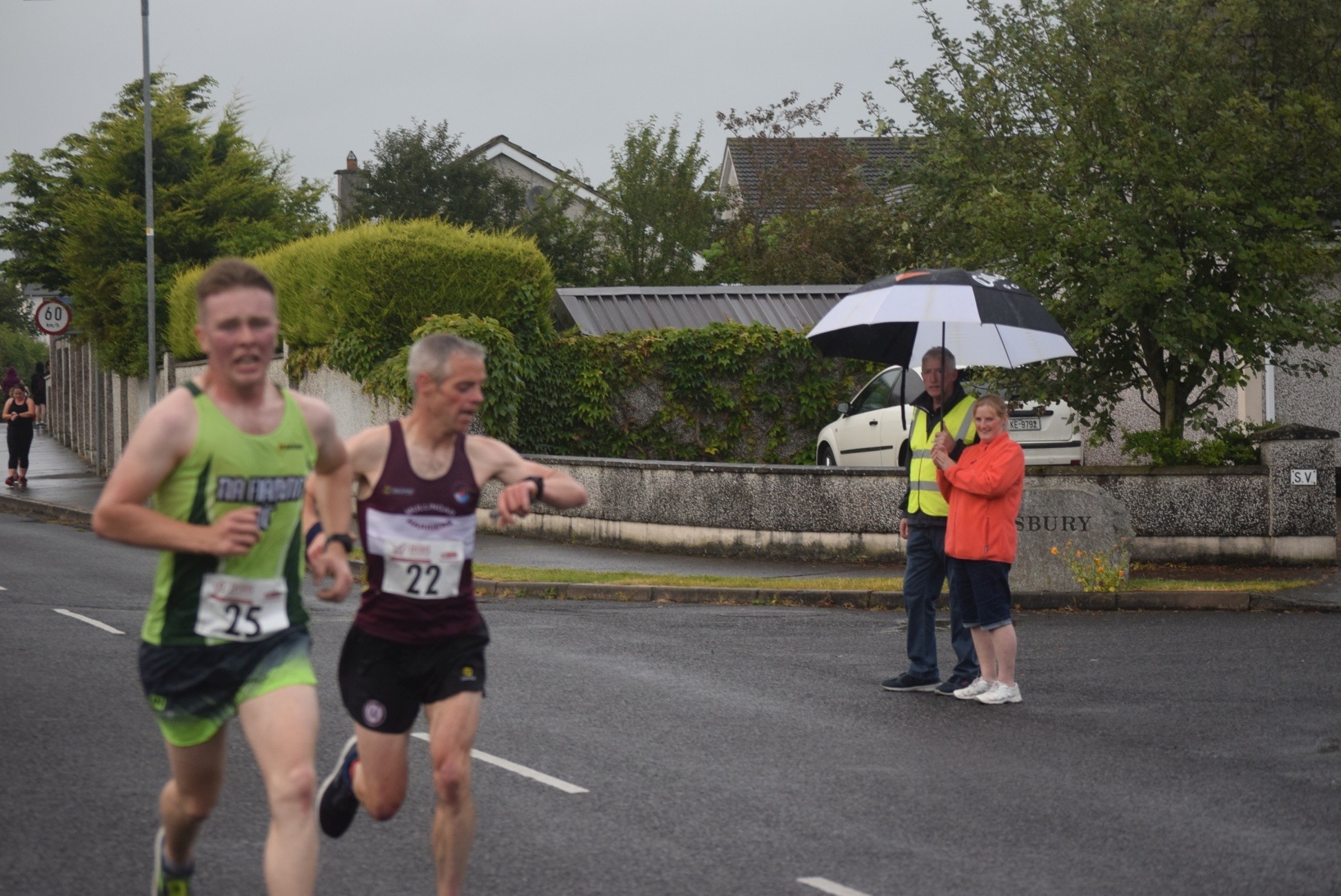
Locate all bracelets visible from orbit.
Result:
[326,533,353,554]
[305,522,322,548]
[524,476,543,498]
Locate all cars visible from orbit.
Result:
[815,357,1086,469]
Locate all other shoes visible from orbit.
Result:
[881,671,941,692]
[954,677,992,699]
[18,476,27,485]
[934,676,972,695]
[151,826,195,896]
[34,422,46,429]
[317,732,364,839]
[974,681,1023,704]
[5,474,19,485]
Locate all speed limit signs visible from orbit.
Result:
[35,299,72,335]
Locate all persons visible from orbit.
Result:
[882,344,981,696]
[2,384,34,487]
[0,359,51,429]
[930,394,1025,704]
[91,259,355,896]
[302,332,588,896]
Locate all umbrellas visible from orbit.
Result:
[805,266,1086,431]
